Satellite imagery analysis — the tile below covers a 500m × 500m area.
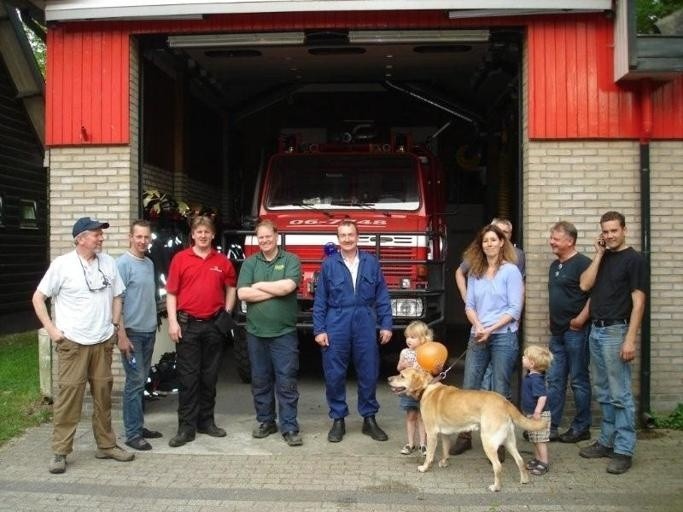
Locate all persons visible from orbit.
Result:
[31,217,136,474]
[237,219,303,445]
[396,319,432,457]
[520,345,553,475]
[448,225,525,465]
[115,220,162,451]
[311,221,393,443]
[580,212,646,475]
[454,215,525,392]
[523,220,591,443]
[164,216,237,448]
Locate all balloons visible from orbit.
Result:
[415,341,449,371]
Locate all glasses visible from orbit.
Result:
[83,270,108,292]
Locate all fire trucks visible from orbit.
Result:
[217,107,452,386]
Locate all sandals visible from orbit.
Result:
[531,461,550,475]
[525,459,538,469]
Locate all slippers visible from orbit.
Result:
[126,440,151,450]
[141,428,162,439]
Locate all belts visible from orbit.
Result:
[594,318,627,327]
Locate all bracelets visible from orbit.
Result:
[224,309,232,315]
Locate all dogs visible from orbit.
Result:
[388,366,548,492]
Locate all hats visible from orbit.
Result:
[71,217,110,238]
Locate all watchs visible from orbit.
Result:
[112,323,120,329]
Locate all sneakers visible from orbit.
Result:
[523,430,558,440]
[48,456,66,473]
[419,442,427,456]
[283,430,303,446]
[401,444,416,455]
[198,425,226,438]
[449,436,471,456]
[94,445,134,462]
[560,428,591,444]
[578,442,612,459]
[489,444,506,464]
[170,431,196,448]
[252,421,278,438]
[606,453,632,474]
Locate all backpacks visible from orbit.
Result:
[159,350,179,392]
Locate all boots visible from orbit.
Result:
[362,415,387,441]
[328,418,346,442]
